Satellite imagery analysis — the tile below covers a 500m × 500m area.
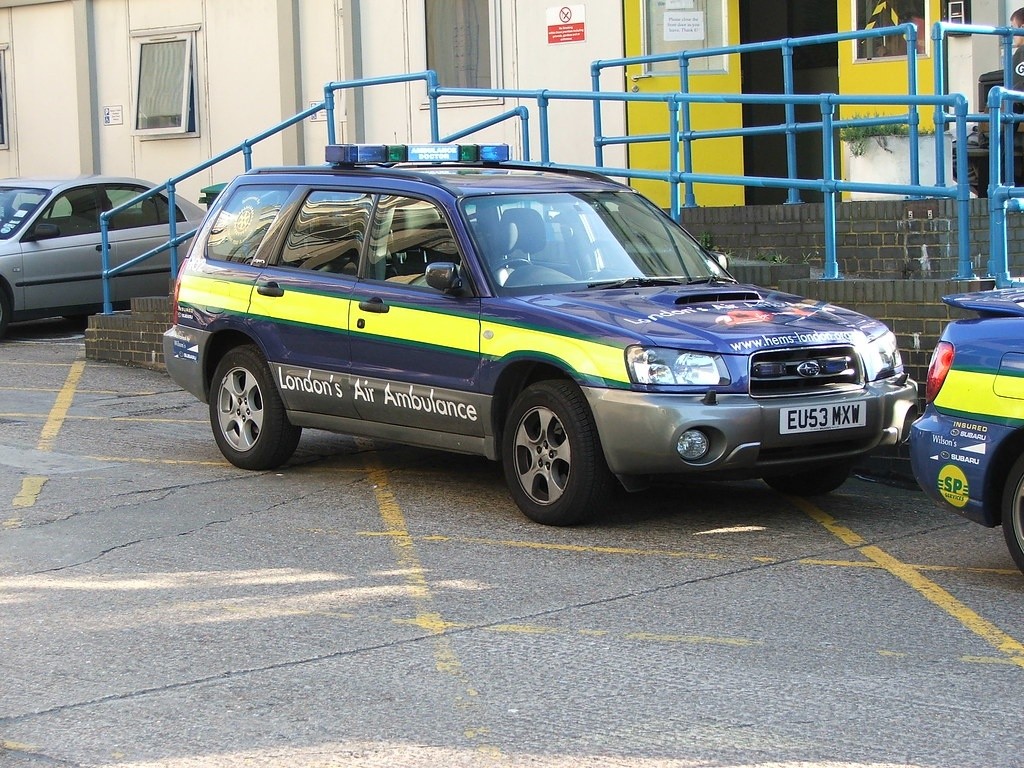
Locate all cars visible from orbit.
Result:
[0,174,210,333]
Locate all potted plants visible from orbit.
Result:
[836,111,953,201]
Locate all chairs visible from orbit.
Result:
[142,197,168,213]
[388,207,441,250]
[18,203,44,218]
[491,208,546,253]
[70,208,97,226]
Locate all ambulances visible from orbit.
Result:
[906,287,1024,575]
[162,144,920,527]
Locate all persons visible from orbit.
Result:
[978,6,1024,198]
